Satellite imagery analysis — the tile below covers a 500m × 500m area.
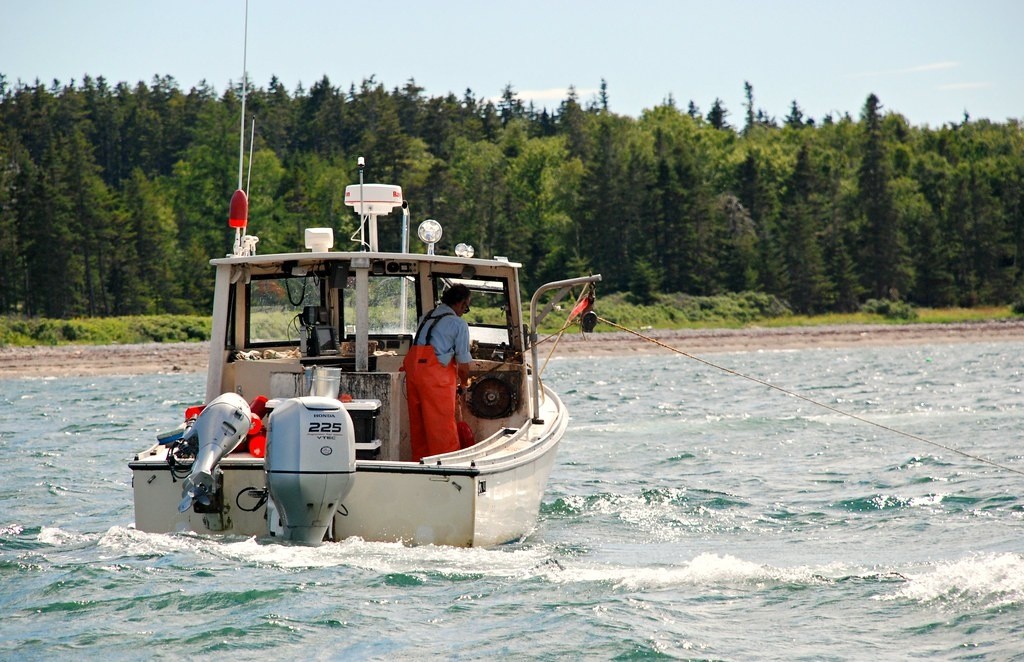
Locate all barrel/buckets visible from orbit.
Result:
[302,365,341,399]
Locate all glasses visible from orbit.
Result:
[465,301,470,314]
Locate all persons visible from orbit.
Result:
[403,283,473,459]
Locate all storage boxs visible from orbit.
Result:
[266,399,382,439]
[355,439,382,461]
